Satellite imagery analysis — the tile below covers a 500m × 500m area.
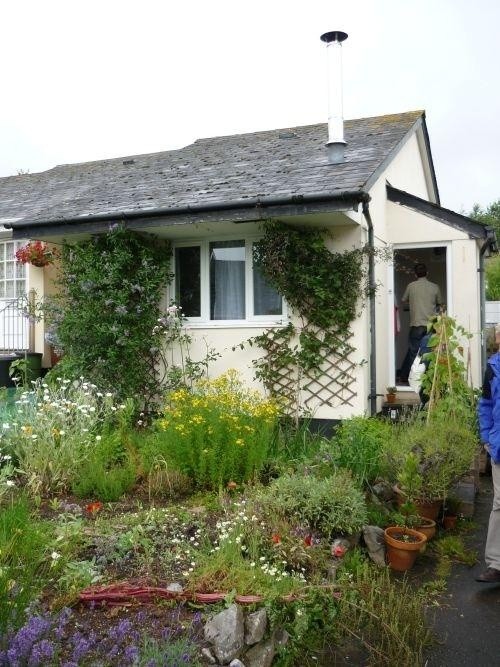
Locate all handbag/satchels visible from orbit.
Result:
[408,349,427,393]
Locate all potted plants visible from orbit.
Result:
[386,385,399,403]
[385,428,476,571]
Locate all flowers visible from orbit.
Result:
[16,239,57,259]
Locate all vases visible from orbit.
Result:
[32,260,47,267]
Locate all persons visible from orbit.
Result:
[400,263,442,383]
[476,347,500,582]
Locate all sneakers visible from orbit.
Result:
[475,567,500,582]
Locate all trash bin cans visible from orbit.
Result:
[0,351,43,391]
